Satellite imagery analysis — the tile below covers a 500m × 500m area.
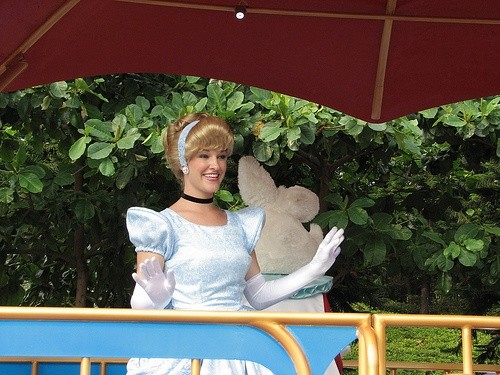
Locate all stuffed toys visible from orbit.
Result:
[236,156,324,312]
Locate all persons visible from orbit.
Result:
[126,113,345,375]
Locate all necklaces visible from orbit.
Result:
[181,192,214,204]
